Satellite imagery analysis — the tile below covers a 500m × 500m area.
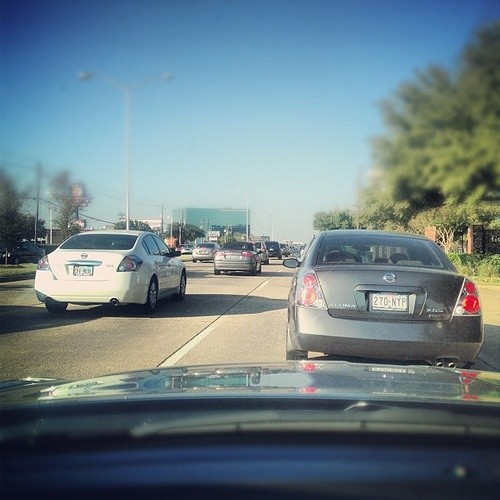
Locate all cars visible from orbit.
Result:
[180,237,303,274]
[283,228,484,368]
[34,229,188,313]
